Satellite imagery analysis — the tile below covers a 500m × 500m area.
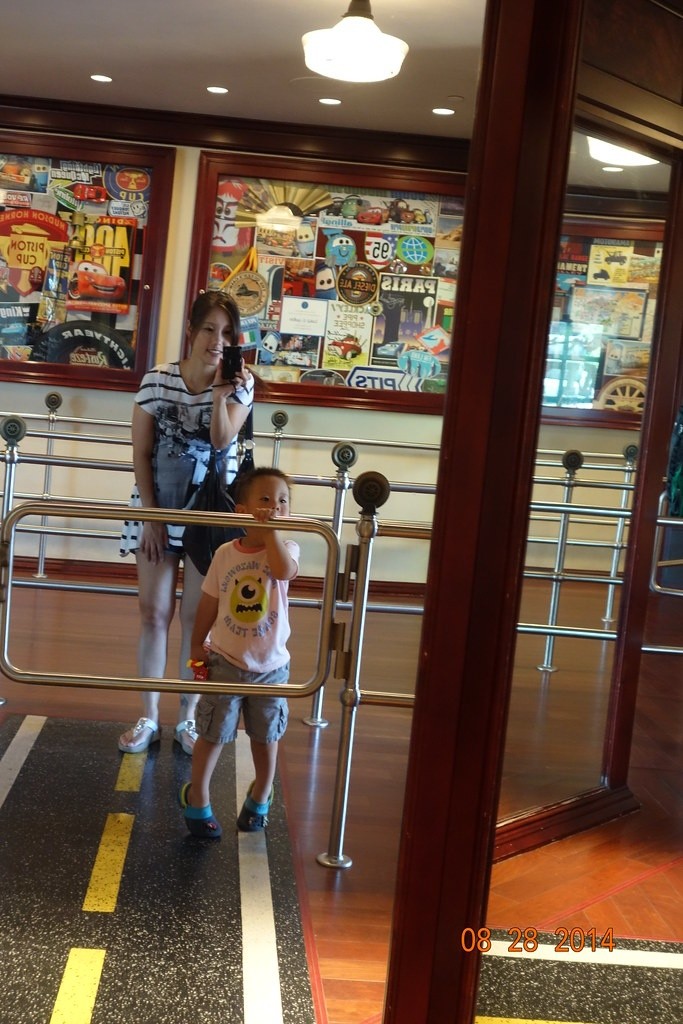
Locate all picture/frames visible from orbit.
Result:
[194,149,467,417]
[539,209,668,430]
[0,128,177,393]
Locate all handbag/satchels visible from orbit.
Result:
[181,404,255,575]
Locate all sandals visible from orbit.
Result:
[236,779,277,833]
[177,779,224,837]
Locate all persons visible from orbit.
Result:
[176,466,302,846]
[118,291,255,756]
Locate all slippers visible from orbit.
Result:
[118,716,199,754]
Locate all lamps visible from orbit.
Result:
[300,0,410,84]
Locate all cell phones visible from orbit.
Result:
[221,345,243,379]
